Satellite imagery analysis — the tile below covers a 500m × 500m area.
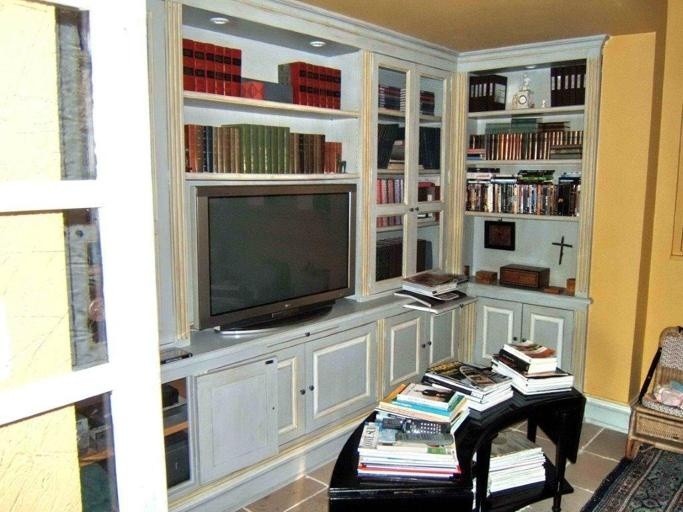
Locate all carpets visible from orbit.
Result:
[579,441,683,512]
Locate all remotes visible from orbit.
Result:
[403,419,451,436]
[383,418,403,429]
[396,433,453,445]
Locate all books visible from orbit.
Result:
[358,338,575,509]
[379,86,406,111]
[380,179,440,226]
[394,273,477,309]
[419,90,435,116]
[470,75,507,111]
[468,118,583,161]
[378,237,425,280]
[551,66,585,107]
[182,37,349,174]
[466,168,582,217]
[379,123,441,170]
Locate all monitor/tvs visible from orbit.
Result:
[194,184,357,334]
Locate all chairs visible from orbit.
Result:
[625,326,683,461]
